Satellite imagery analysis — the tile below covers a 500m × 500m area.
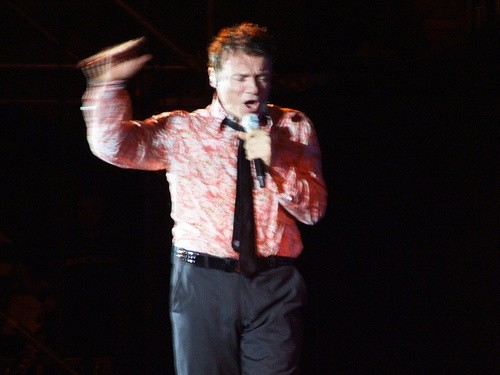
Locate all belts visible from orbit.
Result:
[174,250,296,274]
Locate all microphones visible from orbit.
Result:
[241,112,268,190]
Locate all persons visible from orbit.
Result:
[78,22,328,375]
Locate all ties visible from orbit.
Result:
[224,118,259,276]
